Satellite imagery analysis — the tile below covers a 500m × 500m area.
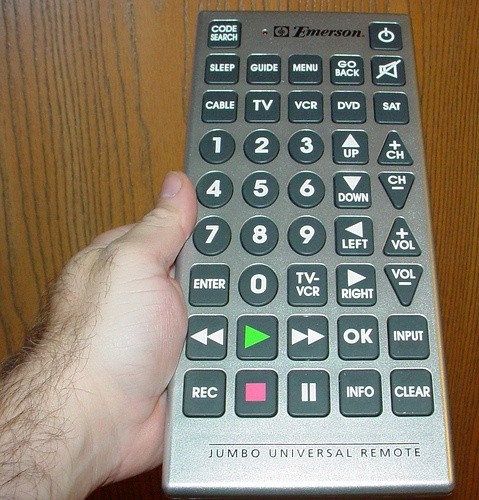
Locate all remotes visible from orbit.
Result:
[160,10,456,495]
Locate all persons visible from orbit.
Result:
[0,171,198,500]
